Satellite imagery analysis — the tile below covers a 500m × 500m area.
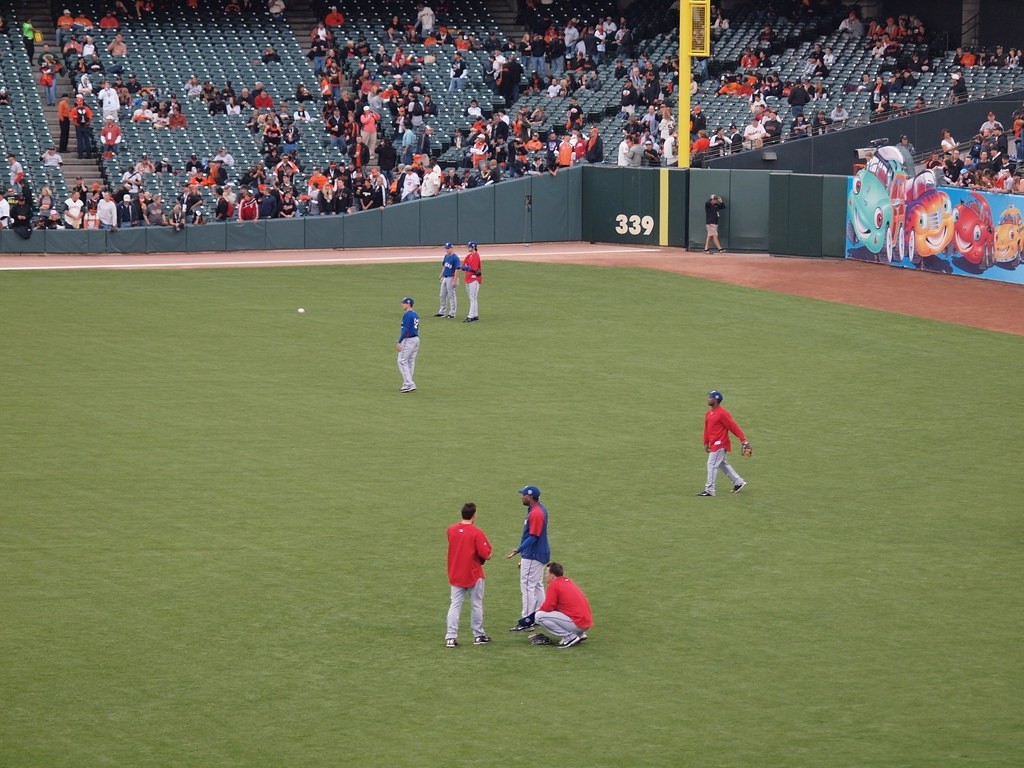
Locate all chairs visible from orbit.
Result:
[1,1,1024,223]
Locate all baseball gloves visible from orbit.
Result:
[528,633,551,645]
[741,442,752,457]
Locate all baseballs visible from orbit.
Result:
[297,308,305,314]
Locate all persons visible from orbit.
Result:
[704,195,727,254]
[504,485,550,633]
[689,0,1024,195]
[0,0,679,232]
[445,503,493,648]
[697,391,752,497]
[435,242,461,318]
[461,241,483,322]
[535,562,592,649]
[397,297,421,393]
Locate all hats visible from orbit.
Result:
[518,486,540,500]
[123,194,131,202]
[994,127,1003,131]
[401,297,413,305]
[444,243,453,248]
[477,134,485,139]
[707,390,722,402]
[467,242,476,248]
[973,134,983,139]
[76,175,82,180]
[404,164,411,171]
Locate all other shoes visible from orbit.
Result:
[435,313,443,316]
[446,315,455,318]
[703,250,713,255]
[463,315,479,322]
[399,386,416,392]
[719,249,727,253]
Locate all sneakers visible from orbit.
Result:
[556,634,580,649]
[580,634,588,639]
[731,480,746,494]
[510,624,534,633]
[446,638,456,647]
[698,491,711,497]
[474,636,491,644]
